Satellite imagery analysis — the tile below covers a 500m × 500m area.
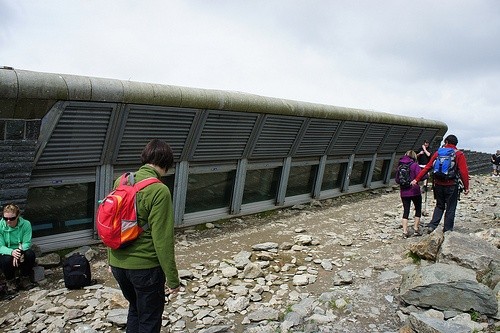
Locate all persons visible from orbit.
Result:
[491,150,500,175]
[410,135,469,232]
[396,150,430,237]
[0,204,33,293]
[108,139,181,333]
[417,139,433,192]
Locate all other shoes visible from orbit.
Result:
[414,229,423,237]
[427,227,434,233]
[403,231,408,239]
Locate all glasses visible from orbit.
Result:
[3,214,17,221]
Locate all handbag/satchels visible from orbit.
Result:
[59,252,98,290]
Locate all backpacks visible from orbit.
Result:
[432,147,460,181]
[398,163,413,188]
[96,172,163,250]
